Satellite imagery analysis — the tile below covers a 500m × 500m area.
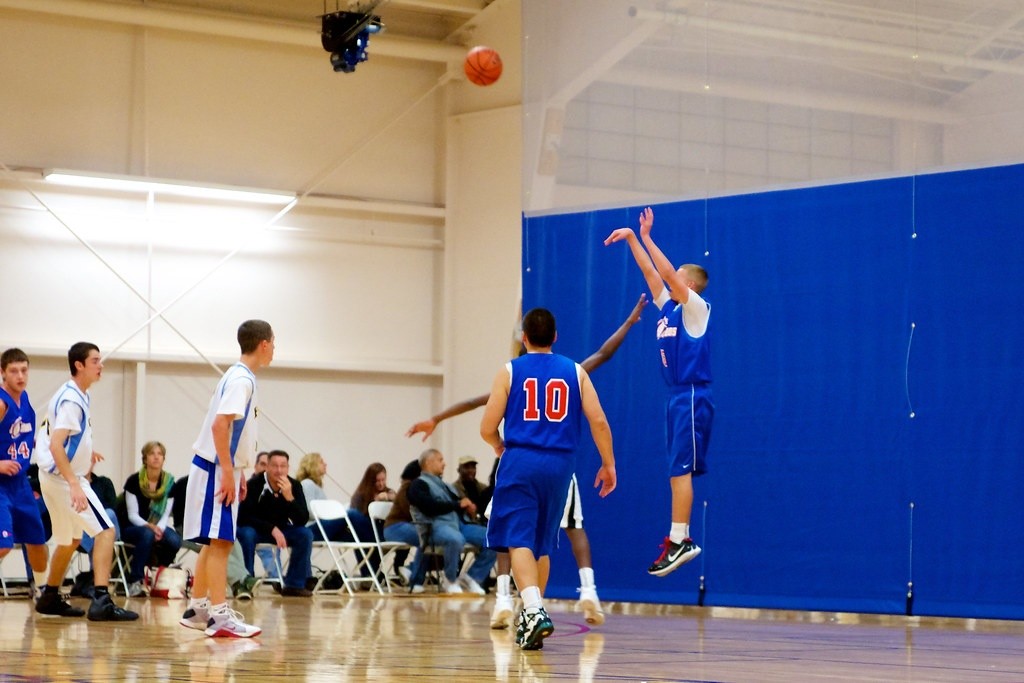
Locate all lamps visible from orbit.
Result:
[315,0,386,75]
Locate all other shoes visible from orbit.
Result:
[404,586,426,594]
[439,572,463,595]
[282,588,312,597]
[32,586,70,603]
[359,581,371,590]
[461,574,486,596]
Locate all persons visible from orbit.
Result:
[384,459,429,593]
[480,307,617,649]
[76,464,120,597]
[405,293,649,629]
[452,457,491,594]
[349,461,403,588]
[179,319,276,637]
[407,449,497,594]
[123,441,182,597]
[297,452,370,590]
[250,451,282,589]
[236,449,312,596]
[604,206,713,578]
[36,341,140,621]
[173,474,263,599]
[0,347,50,604]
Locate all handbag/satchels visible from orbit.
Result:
[144,566,188,599]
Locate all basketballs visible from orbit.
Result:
[464,45,502,87]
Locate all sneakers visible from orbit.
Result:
[648,536,695,575]
[204,603,262,638]
[244,575,263,593]
[513,607,554,650]
[87,595,139,621]
[579,589,604,627]
[491,629,512,650]
[179,601,210,631]
[35,594,84,616]
[658,538,701,577]
[233,587,251,600]
[491,597,513,629]
[129,581,146,597]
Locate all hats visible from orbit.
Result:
[458,455,478,465]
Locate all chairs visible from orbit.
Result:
[110,542,135,597]
[307,499,498,599]
[109,540,130,599]
[0,543,28,599]
[251,543,285,595]
